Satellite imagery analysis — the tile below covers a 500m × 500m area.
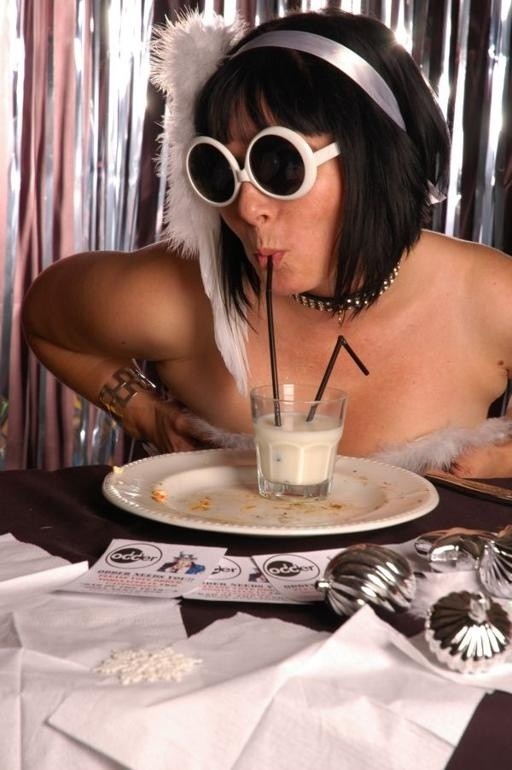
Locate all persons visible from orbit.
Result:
[157,550,205,576]
[21,13,512,481]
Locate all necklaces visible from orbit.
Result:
[289,253,401,328]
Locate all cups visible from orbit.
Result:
[248,382,348,501]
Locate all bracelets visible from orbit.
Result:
[95,356,157,426]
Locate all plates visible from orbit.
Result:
[101,445,442,540]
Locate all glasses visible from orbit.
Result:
[185,124,341,207]
